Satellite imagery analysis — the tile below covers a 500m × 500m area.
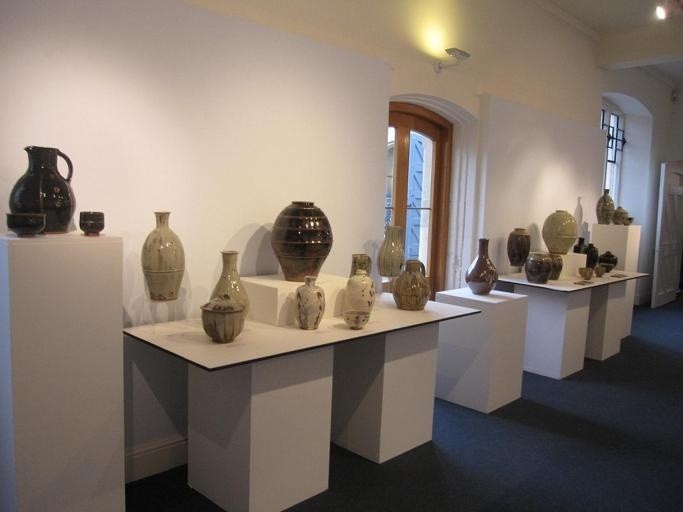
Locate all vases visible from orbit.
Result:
[599,251,617,272]
[11,145,77,233]
[596,189,615,226]
[394,260,431,310]
[583,244,598,269]
[525,252,552,284]
[142,211,186,301]
[271,200,333,280]
[507,228,529,265]
[344,268,372,330]
[465,239,498,298]
[574,235,588,254]
[378,224,404,276]
[550,254,561,280]
[295,275,324,331]
[542,211,580,255]
[614,206,628,224]
[215,250,249,323]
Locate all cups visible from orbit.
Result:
[594,266,606,277]
[77,209,105,238]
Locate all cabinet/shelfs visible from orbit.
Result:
[10,233,124,333]
[500,269,629,333]
[439,289,527,331]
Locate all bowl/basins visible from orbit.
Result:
[578,267,593,281]
[621,217,634,226]
[599,263,616,273]
[342,310,370,330]
[3,212,47,238]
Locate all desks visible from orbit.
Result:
[123,294,482,332]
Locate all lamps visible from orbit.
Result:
[433,47,472,73]
